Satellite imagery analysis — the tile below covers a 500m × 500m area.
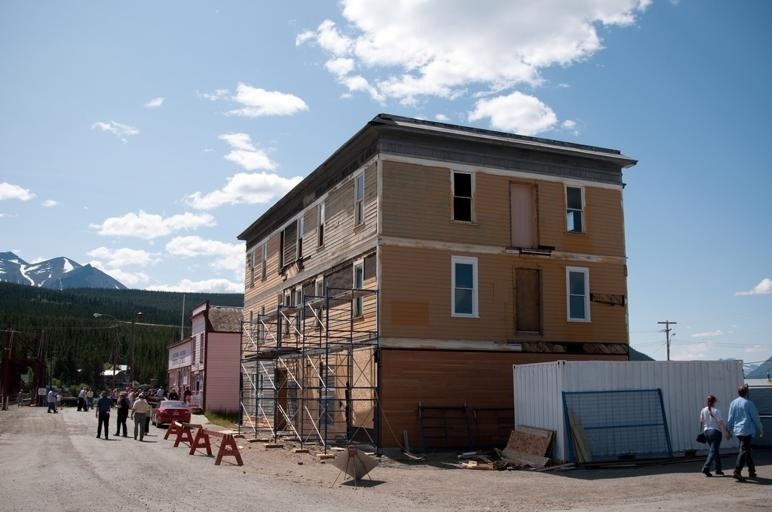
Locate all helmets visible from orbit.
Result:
[738,384,748,395]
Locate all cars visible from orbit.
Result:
[151,399,191,428]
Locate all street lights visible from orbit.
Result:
[92,310,144,386]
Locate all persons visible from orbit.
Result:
[113,391,131,437]
[94,389,113,440]
[17,388,24,408]
[130,393,146,441]
[724,383,764,483]
[144,401,151,435]
[698,394,730,477]
[47,382,191,414]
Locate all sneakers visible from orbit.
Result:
[733,471,756,481]
[703,469,724,477]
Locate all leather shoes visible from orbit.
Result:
[113,434,127,437]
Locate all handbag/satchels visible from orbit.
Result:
[696,433,706,443]
